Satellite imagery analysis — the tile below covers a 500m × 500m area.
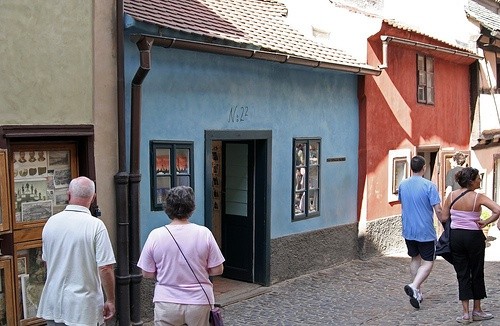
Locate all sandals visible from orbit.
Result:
[456,315,470,324]
[472,311,493,321]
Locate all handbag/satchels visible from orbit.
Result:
[209,306,223,325]
[435,220,452,265]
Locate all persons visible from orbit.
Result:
[441,167,500,323]
[445,152,465,200]
[137,186,225,326]
[399,156,443,308]
[19,152,26,163]
[36,176,116,326]
[29,151,36,162]
[38,151,45,161]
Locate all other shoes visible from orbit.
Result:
[404,282,421,308]
[417,291,423,302]
